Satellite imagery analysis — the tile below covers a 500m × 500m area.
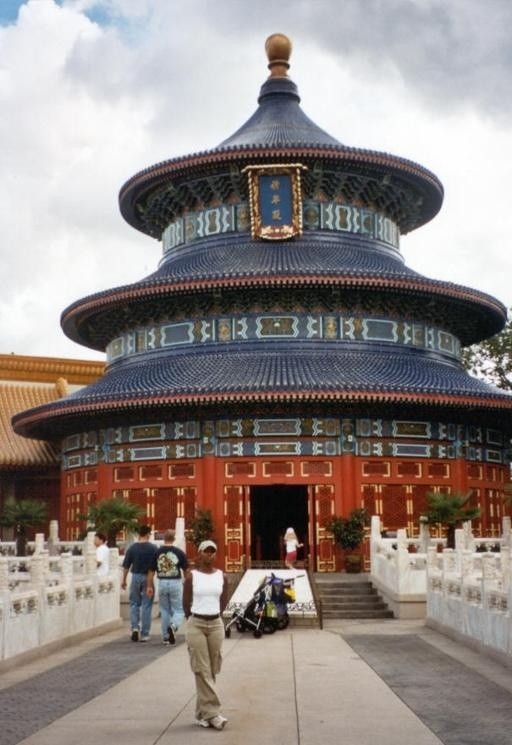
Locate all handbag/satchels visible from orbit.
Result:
[266,601,278,619]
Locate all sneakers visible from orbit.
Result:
[167,625,176,644]
[163,639,170,645]
[208,715,228,731]
[139,636,151,642]
[195,719,210,728]
[131,628,139,642]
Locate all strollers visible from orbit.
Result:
[224,570,307,638]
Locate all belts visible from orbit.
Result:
[190,613,219,621]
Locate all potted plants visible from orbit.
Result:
[324,506,371,576]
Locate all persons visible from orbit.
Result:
[282,527,304,569]
[121,524,159,642]
[94,531,110,576]
[181,539,229,731]
[145,529,190,646]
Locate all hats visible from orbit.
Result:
[286,527,294,533]
[198,540,218,552]
